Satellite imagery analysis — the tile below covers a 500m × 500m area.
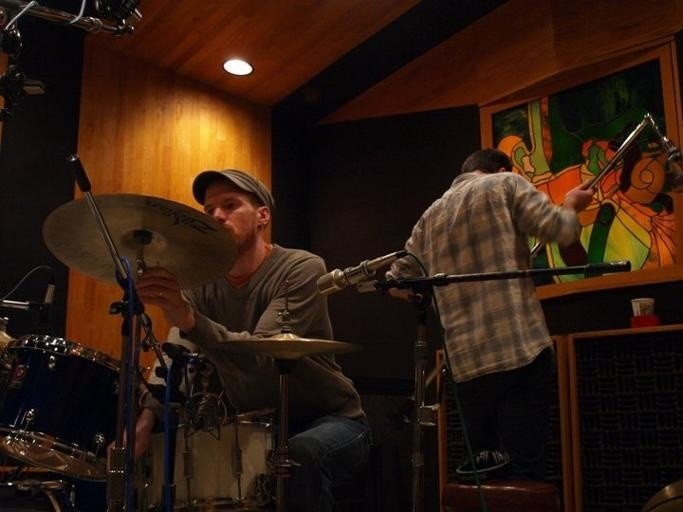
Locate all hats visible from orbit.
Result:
[192,169,275,210]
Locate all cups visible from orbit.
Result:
[631,298,655,316]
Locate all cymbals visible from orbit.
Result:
[42,194,239,289]
[204,333,356,360]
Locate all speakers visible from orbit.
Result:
[568,324,683,512]
[436,335,574,512]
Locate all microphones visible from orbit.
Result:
[38,279,55,327]
[317,249,405,297]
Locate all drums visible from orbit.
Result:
[1,453,72,491]
[0,334,121,482]
[132,415,277,511]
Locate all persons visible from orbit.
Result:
[106,169,377,509]
[385,149,597,478]
